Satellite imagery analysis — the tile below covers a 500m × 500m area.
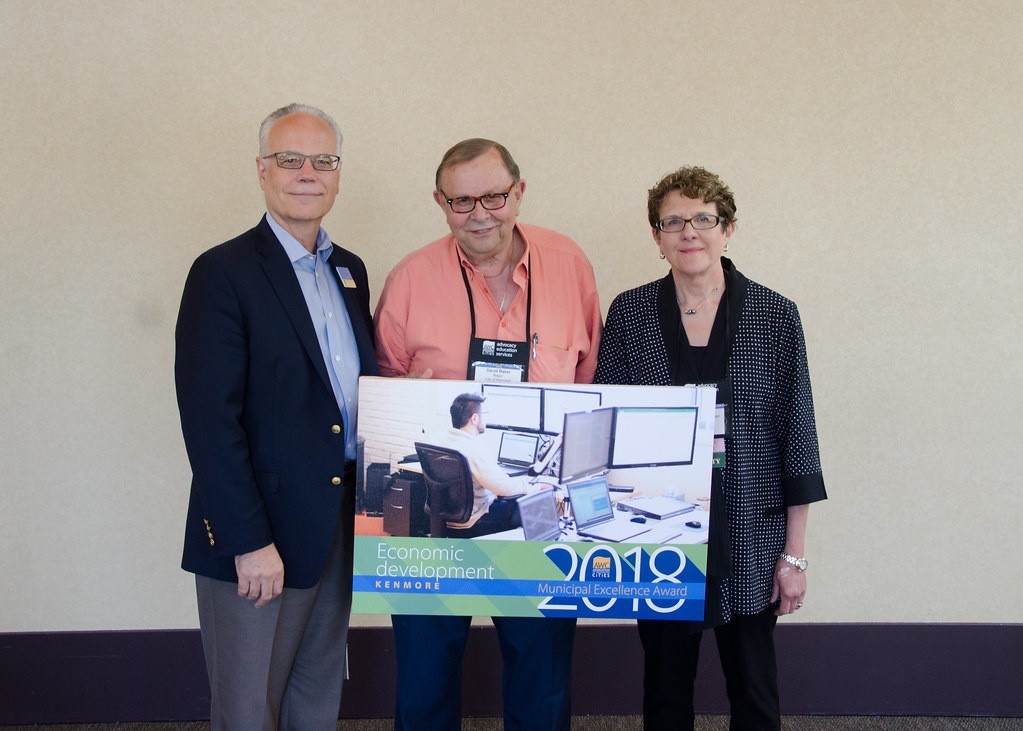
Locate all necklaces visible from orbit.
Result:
[499,234,516,312]
[674,283,721,315]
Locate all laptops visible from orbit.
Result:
[566,476,652,543]
[497,433,539,477]
[515,487,589,542]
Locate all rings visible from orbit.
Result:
[797,603,803,607]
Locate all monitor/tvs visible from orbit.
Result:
[482,384,700,502]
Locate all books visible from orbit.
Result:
[616,494,695,519]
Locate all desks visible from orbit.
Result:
[394,461,709,544]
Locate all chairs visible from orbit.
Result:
[412,442,525,537]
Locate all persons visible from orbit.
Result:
[371,138,606,731]
[593,165,829,731]
[429,392,564,538]
[174,104,379,731]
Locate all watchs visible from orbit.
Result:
[780,552,808,571]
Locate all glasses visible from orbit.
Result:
[655,214,725,233]
[441,181,517,213]
[260,151,341,171]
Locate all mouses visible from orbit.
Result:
[581,538,593,542]
[685,521,702,528]
[630,514,647,523]
